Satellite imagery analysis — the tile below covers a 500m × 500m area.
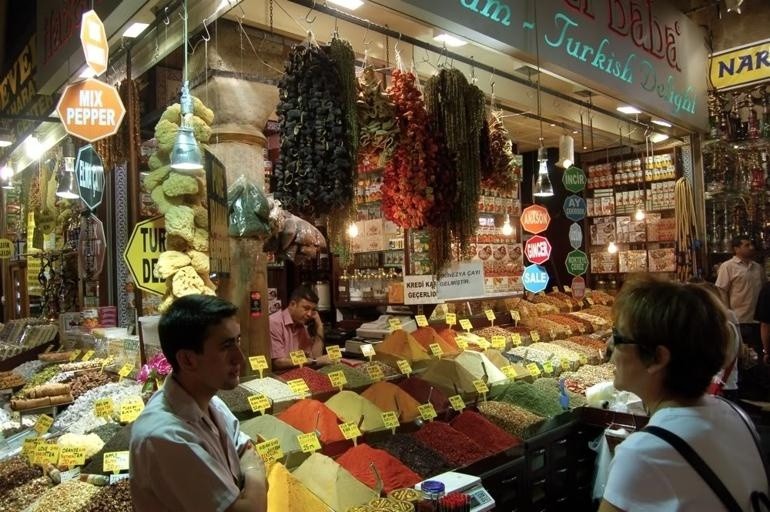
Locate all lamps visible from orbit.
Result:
[651,118,671,128]
[169,0,204,171]
[122,10,156,38]
[635,208,644,220]
[433,28,466,48]
[0,134,14,147]
[617,102,641,115]
[328,0,364,12]
[553,134,575,170]
[1,148,15,191]
[54,0,80,200]
[608,242,617,253]
[532,0,556,197]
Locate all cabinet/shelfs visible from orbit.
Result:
[580,137,682,289]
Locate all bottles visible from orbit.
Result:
[311,280,330,311]
[339,267,403,303]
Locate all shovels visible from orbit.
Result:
[481,362,488,384]
[423,386,433,405]
[516,348,527,368]
[369,462,383,497]
[393,394,402,420]
[559,379,568,410]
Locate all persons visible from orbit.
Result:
[268,286,340,370]
[693,280,740,405]
[597,277,767,512]
[129,293,270,511]
[753,280,770,403]
[715,234,767,401]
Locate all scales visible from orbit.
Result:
[415,471,496,512]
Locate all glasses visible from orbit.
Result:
[611,321,650,346]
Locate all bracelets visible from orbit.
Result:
[763,348,767,353]
[310,356,317,369]
[244,466,266,477]
